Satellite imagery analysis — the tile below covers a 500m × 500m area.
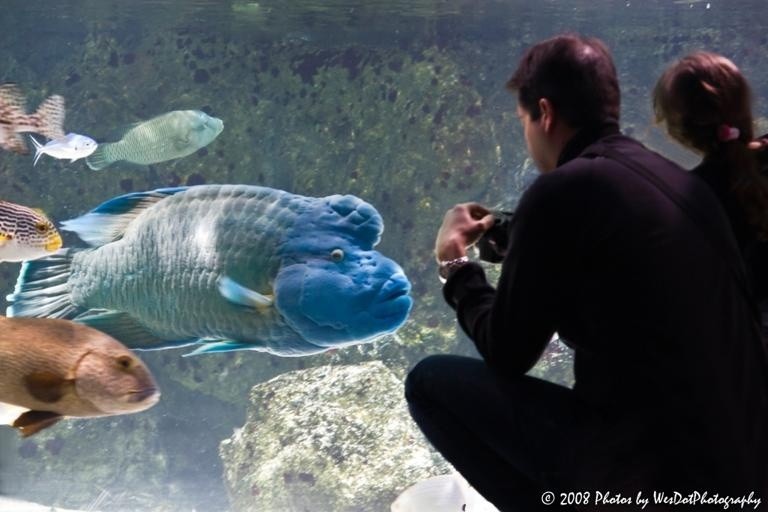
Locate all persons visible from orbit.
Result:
[404,36,768,512]
[648,52,768,338]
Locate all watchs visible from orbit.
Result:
[437,255,470,285]
